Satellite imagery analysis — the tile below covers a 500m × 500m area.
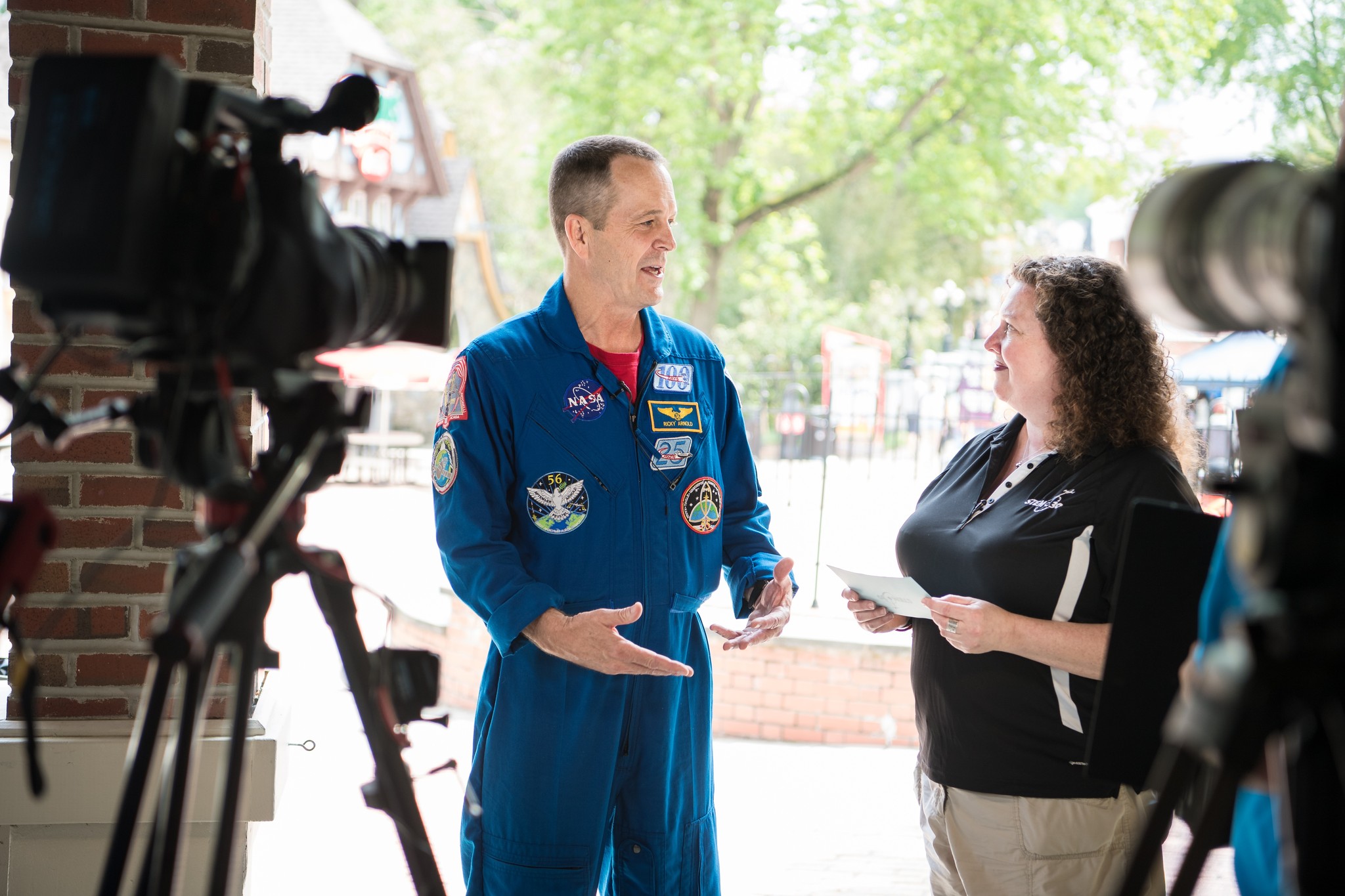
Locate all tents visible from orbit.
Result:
[1163,329,1286,387]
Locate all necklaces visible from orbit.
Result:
[1016,436,1059,468]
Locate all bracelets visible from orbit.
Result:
[748,579,773,608]
[895,625,912,632]
[900,617,912,628]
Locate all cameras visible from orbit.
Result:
[1126,162,1345,345]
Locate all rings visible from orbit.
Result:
[946,618,959,633]
[750,631,753,633]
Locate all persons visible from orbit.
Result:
[1185,388,1258,482]
[1181,335,1291,896]
[432,134,798,896]
[841,254,1204,896]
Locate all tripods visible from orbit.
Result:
[96,360,448,896]
